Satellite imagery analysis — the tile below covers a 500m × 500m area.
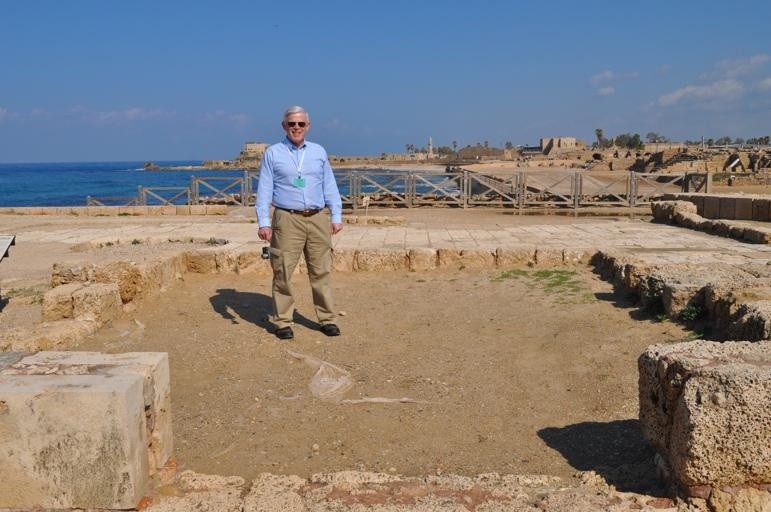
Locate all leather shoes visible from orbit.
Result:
[276,326,295,339]
[321,323,342,337]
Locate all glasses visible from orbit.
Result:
[285,120,309,128]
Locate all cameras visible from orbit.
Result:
[262,245,271,259]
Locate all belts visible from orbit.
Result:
[275,205,325,218]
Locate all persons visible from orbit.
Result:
[253,104,346,340]
